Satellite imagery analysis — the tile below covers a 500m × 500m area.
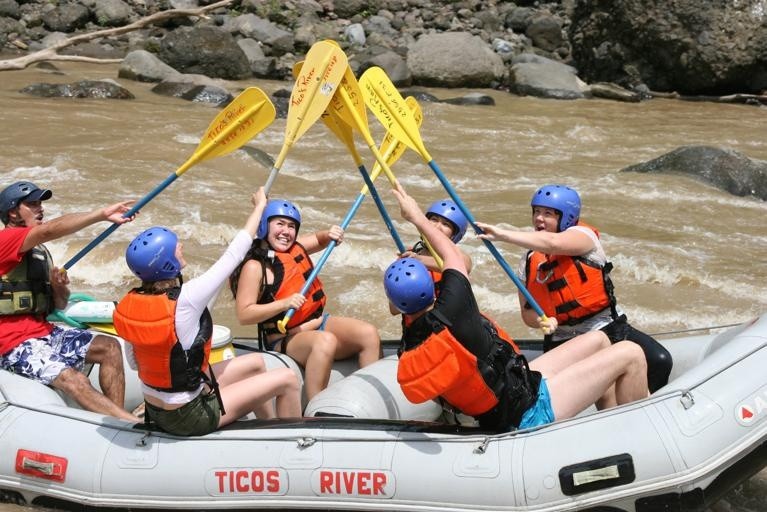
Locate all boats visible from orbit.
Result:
[0,309,767,512]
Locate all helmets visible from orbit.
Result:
[124,227,180,282]
[424,201,466,246]
[0,181,52,223]
[529,182,580,233]
[254,198,302,242]
[382,259,436,314]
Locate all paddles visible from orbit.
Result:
[359,65,558,333]
[292,60,410,258]
[55,87,277,275]
[276,95,424,332]
[323,39,446,272]
[210,40,348,310]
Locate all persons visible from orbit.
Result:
[389,197,472,332]
[473,184,673,394]
[112,182,303,437]
[383,178,649,424]
[0,180,144,425]
[231,198,381,400]
